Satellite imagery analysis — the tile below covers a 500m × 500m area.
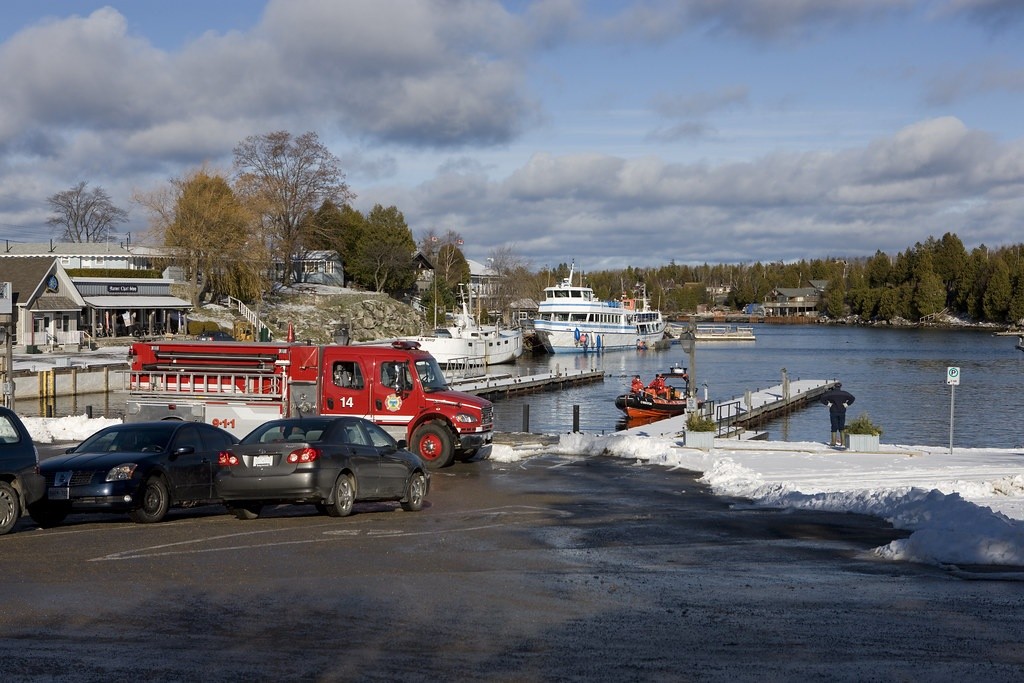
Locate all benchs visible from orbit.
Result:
[299,287,318,294]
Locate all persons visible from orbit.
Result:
[630,374,667,399]
[821,383,855,446]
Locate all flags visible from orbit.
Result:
[458,240,464,245]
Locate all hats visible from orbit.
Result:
[834,383,842,386]
[656,373,662,377]
[636,375,640,379]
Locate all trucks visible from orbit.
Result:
[125,339,495,473]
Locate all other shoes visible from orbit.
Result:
[828,443,845,447]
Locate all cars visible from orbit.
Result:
[28,420,241,524]
[214,414,431,518]
[0,403,41,536]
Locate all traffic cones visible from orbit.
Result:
[286,323,296,343]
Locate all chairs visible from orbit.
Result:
[348,429,359,443]
[382,365,397,388]
[399,367,412,389]
[288,434,305,439]
[306,430,323,439]
[264,432,284,443]
[334,365,361,389]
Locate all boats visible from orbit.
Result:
[390,301,523,365]
[533,260,668,349]
[614,364,705,419]
